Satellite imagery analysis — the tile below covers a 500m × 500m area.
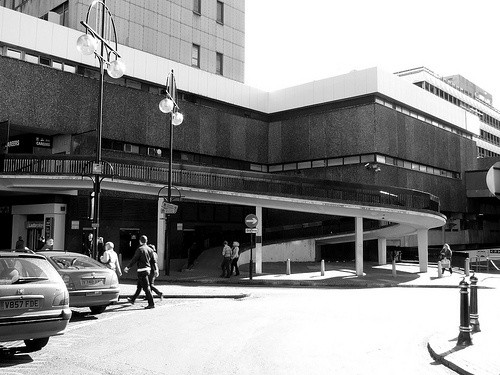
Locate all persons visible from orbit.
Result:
[230,242,240,276]
[83,233,122,276]
[220,241,232,278]
[392,251,402,262]
[15,236,25,252]
[124,235,159,309]
[440,243,453,276]
[143,244,163,301]
[36,236,54,250]
[130,235,137,258]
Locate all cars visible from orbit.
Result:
[0,251,72,351]
[35,251,120,315]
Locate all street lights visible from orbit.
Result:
[77,0,126,262]
[158,68,183,276]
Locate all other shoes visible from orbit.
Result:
[145,304,154,309]
[219,273,240,278]
[127,297,134,304]
[158,293,162,297]
[144,296,148,300]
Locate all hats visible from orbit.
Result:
[46,238,54,246]
[223,241,228,244]
[149,244,156,251]
[105,241,114,248]
[140,235,147,243]
